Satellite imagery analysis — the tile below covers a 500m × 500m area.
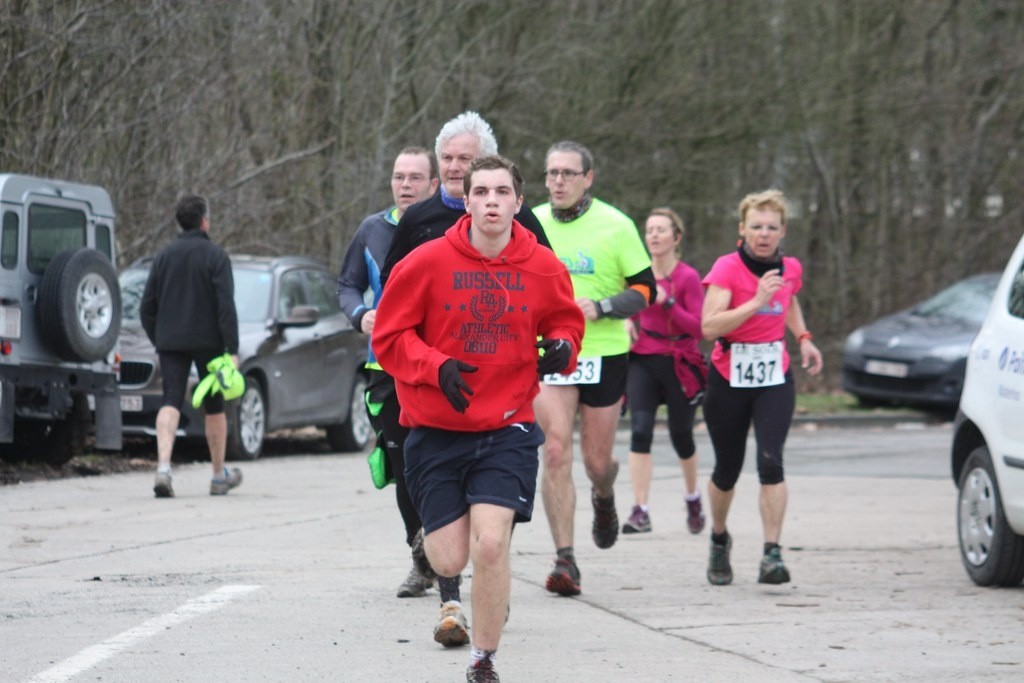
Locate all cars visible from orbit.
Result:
[840,270,1003,419]
[117,247,371,462]
[950,233,1024,587]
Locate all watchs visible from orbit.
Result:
[599,299,614,313]
[665,298,675,308]
[798,333,814,341]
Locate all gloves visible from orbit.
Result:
[533,338,572,374]
[440,357,478,414]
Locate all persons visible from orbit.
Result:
[140,196,241,497]
[379,111,556,599]
[621,208,705,536]
[533,141,657,597]
[334,145,440,488]
[700,190,823,585]
[371,155,585,682]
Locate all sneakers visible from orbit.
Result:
[466,657,498,683]
[707,530,733,585]
[398,568,433,597]
[592,484,620,548]
[210,467,241,494]
[153,473,174,497]
[623,509,650,534]
[685,496,705,535]
[759,558,789,586]
[412,529,439,580]
[433,601,471,648]
[548,562,582,595]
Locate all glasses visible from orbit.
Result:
[543,169,590,183]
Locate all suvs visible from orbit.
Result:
[0,172,126,463]
